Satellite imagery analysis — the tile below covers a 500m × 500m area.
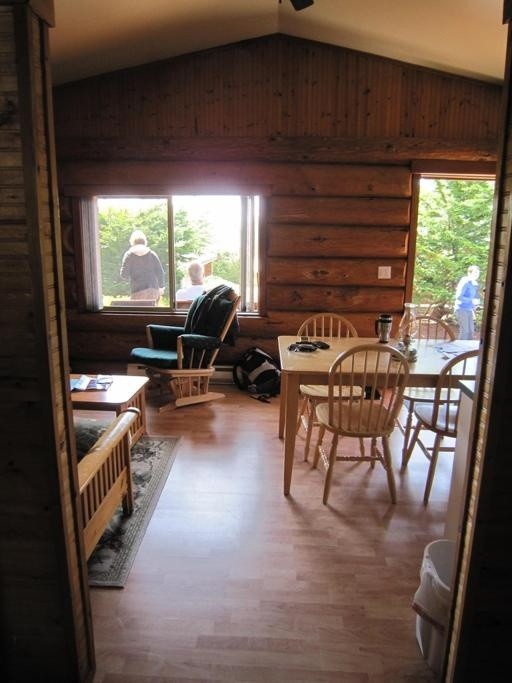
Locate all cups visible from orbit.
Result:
[375,314,393,344]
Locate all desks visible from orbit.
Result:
[277,335,481,497]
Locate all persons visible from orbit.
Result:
[119,229,164,306]
[175,261,206,303]
[453,265,479,340]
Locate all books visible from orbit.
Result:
[70,374,113,392]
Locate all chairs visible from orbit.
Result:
[313,344,410,505]
[297,313,365,462]
[401,349,480,505]
[396,316,457,464]
[126,284,238,414]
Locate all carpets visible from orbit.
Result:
[74,424,185,589]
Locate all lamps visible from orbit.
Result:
[290,0,314,12]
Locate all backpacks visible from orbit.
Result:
[232,344,283,403]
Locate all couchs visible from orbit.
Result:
[78,407,143,563]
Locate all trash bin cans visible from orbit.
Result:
[412,539,459,678]
